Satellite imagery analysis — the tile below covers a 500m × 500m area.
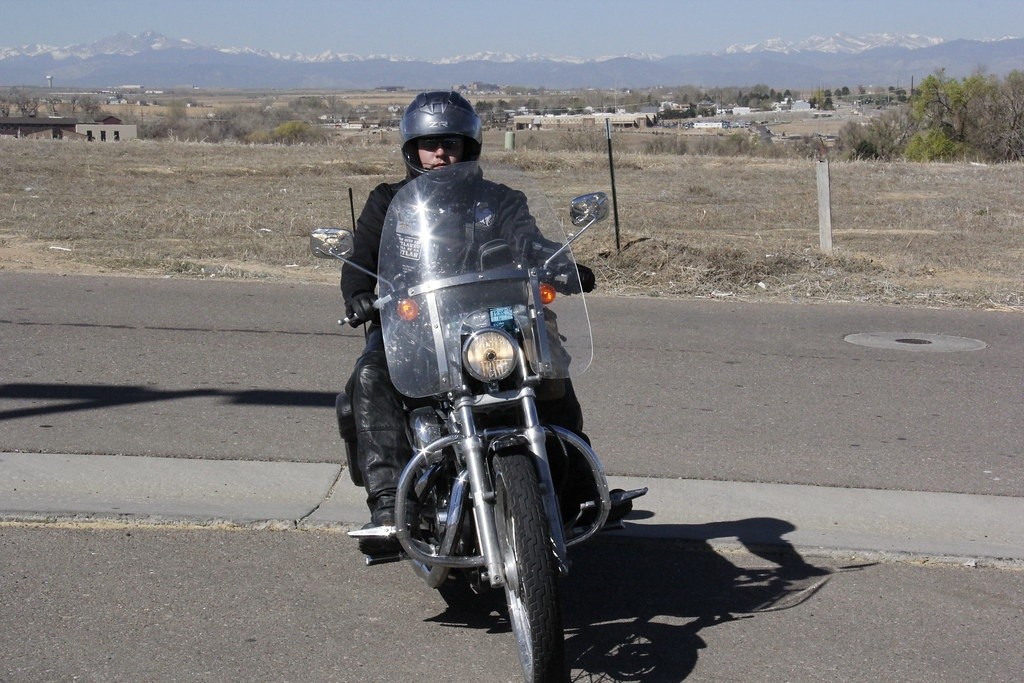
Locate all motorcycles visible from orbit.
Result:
[308,160,649,683]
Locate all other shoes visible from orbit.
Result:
[370,506,417,530]
[576,488,631,526]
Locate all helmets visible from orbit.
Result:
[399,91,483,177]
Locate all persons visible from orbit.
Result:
[340,91,633,556]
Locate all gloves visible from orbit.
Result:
[345,291,379,323]
[551,262,595,296]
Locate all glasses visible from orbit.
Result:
[413,139,461,149]
